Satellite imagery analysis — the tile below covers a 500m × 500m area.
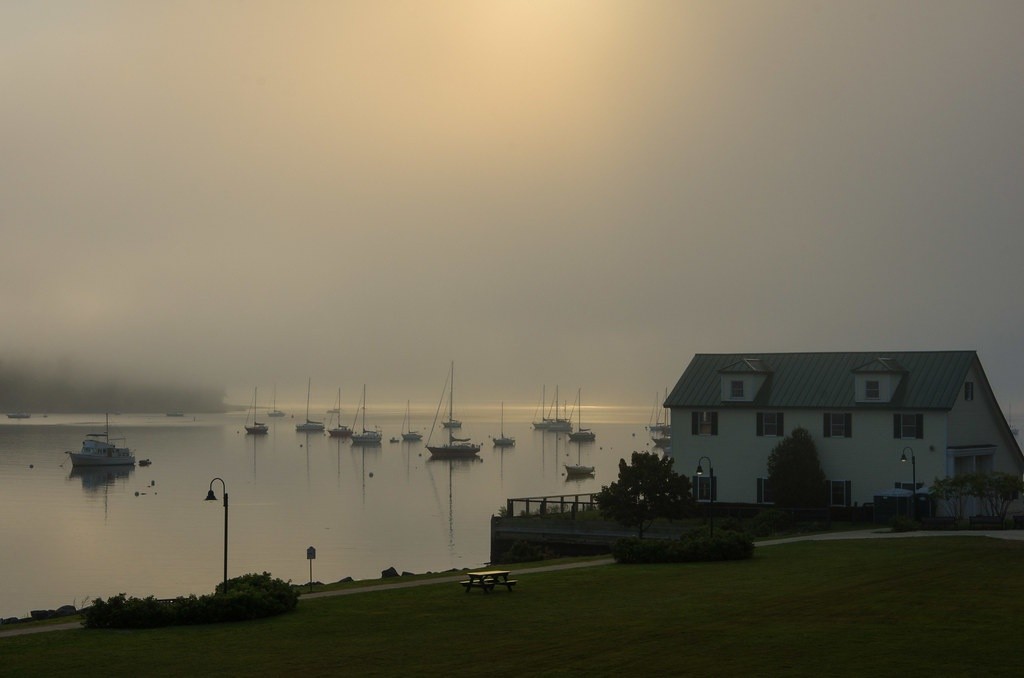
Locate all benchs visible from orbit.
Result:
[459,578,518,590]
[922,516,958,532]
[969,516,1005,530]
[1012,516,1024,530]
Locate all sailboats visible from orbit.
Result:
[648,387,671,451]
[530,384,574,432]
[565,388,596,441]
[563,438,596,476]
[426,362,482,457]
[268,386,286,418]
[296,379,327,431]
[400,400,423,440]
[493,402,516,446]
[327,388,354,437]
[244,388,270,434]
[350,383,383,443]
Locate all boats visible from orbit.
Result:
[6,412,31,418]
[67,411,137,467]
[440,419,463,427]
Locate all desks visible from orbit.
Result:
[464,570,514,595]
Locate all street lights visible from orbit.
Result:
[201,479,227,593]
[901,447,920,523]
[696,456,714,538]
